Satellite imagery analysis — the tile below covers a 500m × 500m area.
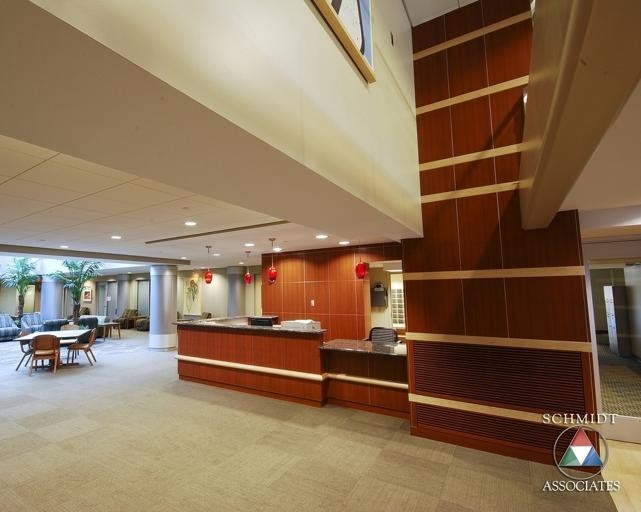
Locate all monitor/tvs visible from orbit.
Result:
[251,317,273,326]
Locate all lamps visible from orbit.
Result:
[205,246,212,284]
[244,251,251,285]
[355,240,366,279]
[267,237,277,285]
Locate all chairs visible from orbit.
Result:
[200,312,211,319]
[0,308,149,376]
[362,327,402,345]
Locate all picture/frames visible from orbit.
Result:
[82,289,92,303]
[313,0,377,84]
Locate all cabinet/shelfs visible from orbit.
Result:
[603,284,631,358]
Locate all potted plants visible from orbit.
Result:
[0,257,44,321]
[49,259,103,325]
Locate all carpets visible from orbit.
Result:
[601,376,641,418]
